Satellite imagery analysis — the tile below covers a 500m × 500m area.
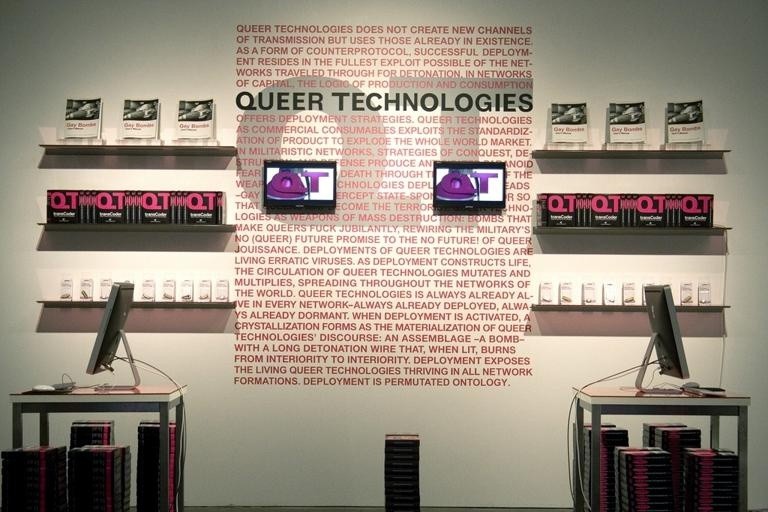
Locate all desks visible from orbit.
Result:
[573,386,751,512]
[9,383,188,512]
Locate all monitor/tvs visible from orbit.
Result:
[262,159,336,208]
[634,284,690,395]
[86,282,140,390]
[432,161,506,210]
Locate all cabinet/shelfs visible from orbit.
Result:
[36,144,236,309]
[532,150,732,312]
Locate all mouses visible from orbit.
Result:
[684,382,698,389]
[32,384,56,391]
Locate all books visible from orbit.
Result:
[65,98,103,139]
[121,99,161,139]
[609,102,646,144]
[178,99,217,139]
[667,101,705,144]
[549,103,588,143]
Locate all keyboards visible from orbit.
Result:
[52,383,75,390]
[684,388,726,397]
